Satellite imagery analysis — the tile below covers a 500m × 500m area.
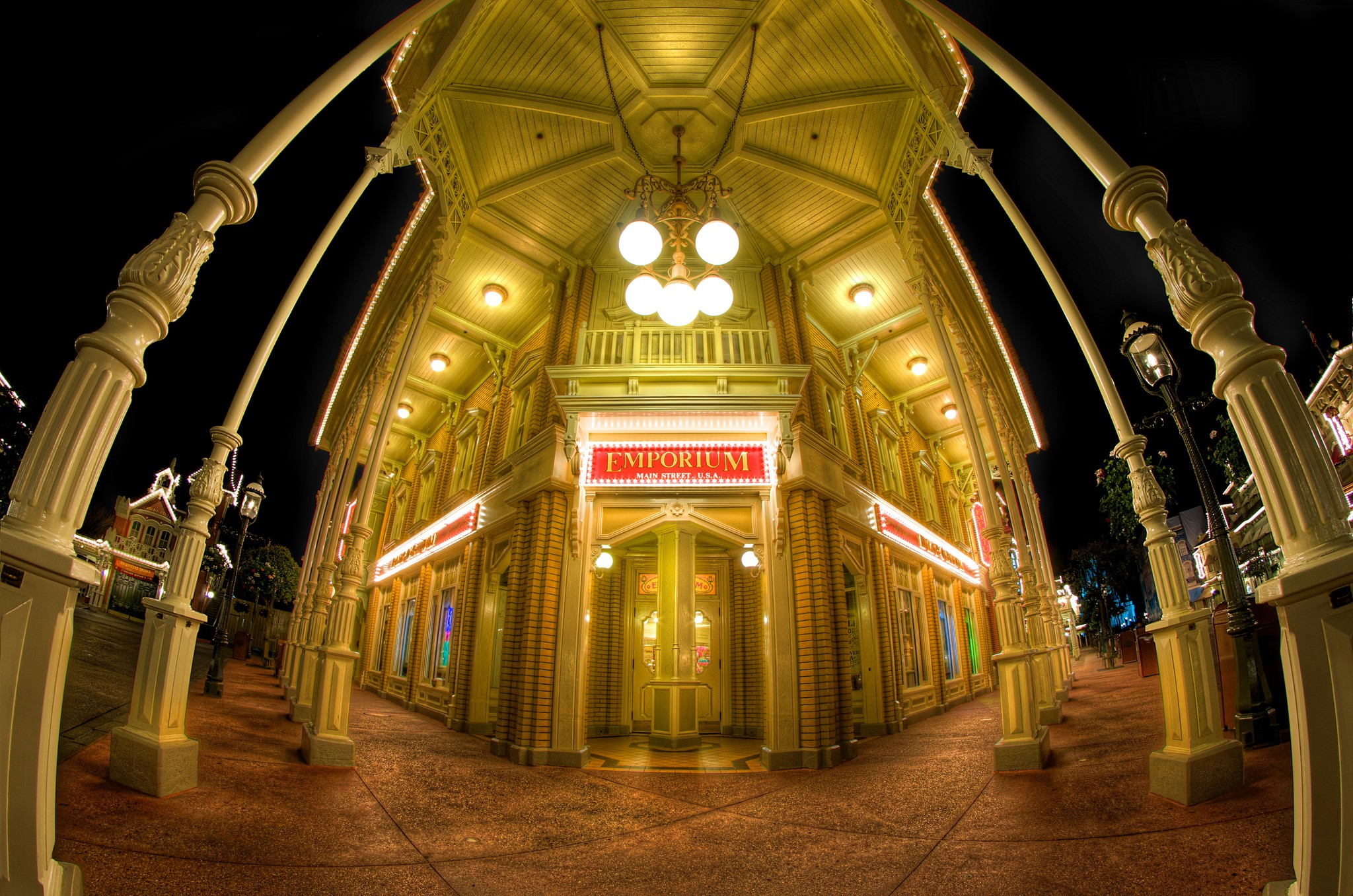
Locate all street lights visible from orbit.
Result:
[203,471,265,699]
[1121,306,1291,752]
